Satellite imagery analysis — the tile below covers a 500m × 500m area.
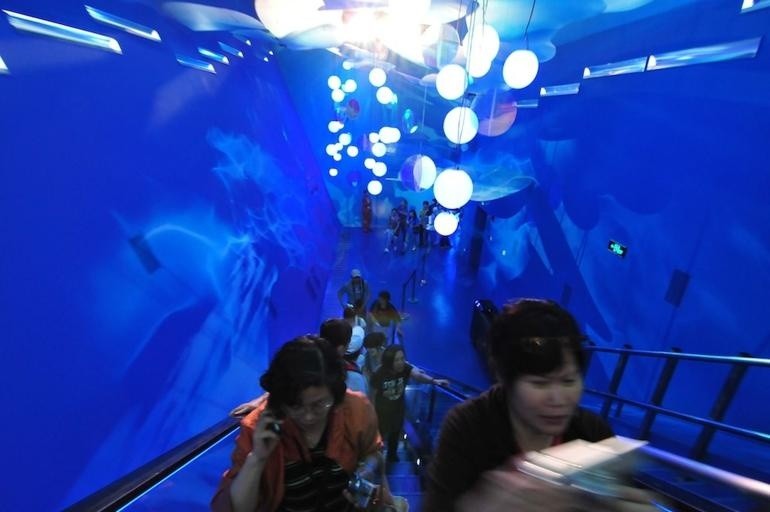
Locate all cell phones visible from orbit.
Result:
[270,413,281,435]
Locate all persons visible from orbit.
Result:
[320,269,449,462]
[210,335,410,512]
[421,298,656,512]
[362,190,446,255]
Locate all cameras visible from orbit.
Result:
[346,473,380,510]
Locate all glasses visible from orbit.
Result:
[520,336,590,353]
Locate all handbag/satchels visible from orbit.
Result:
[384,495,410,512]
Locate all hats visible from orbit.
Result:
[350,269,361,278]
[345,326,365,355]
